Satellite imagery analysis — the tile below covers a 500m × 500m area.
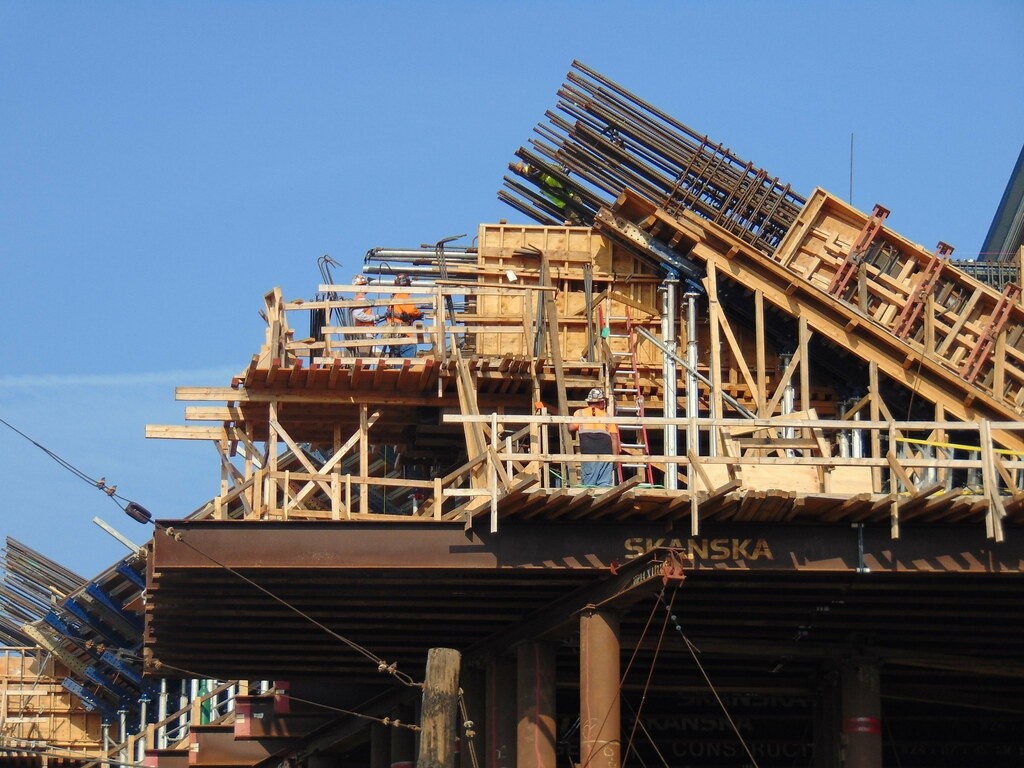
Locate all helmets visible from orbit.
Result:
[353,275,367,286]
[516,161,525,173]
[585,387,607,403]
[395,273,411,286]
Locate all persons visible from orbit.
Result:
[567,388,619,490]
[351,274,380,368]
[512,159,583,226]
[383,273,424,369]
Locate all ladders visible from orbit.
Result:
[596,302,656,489]
